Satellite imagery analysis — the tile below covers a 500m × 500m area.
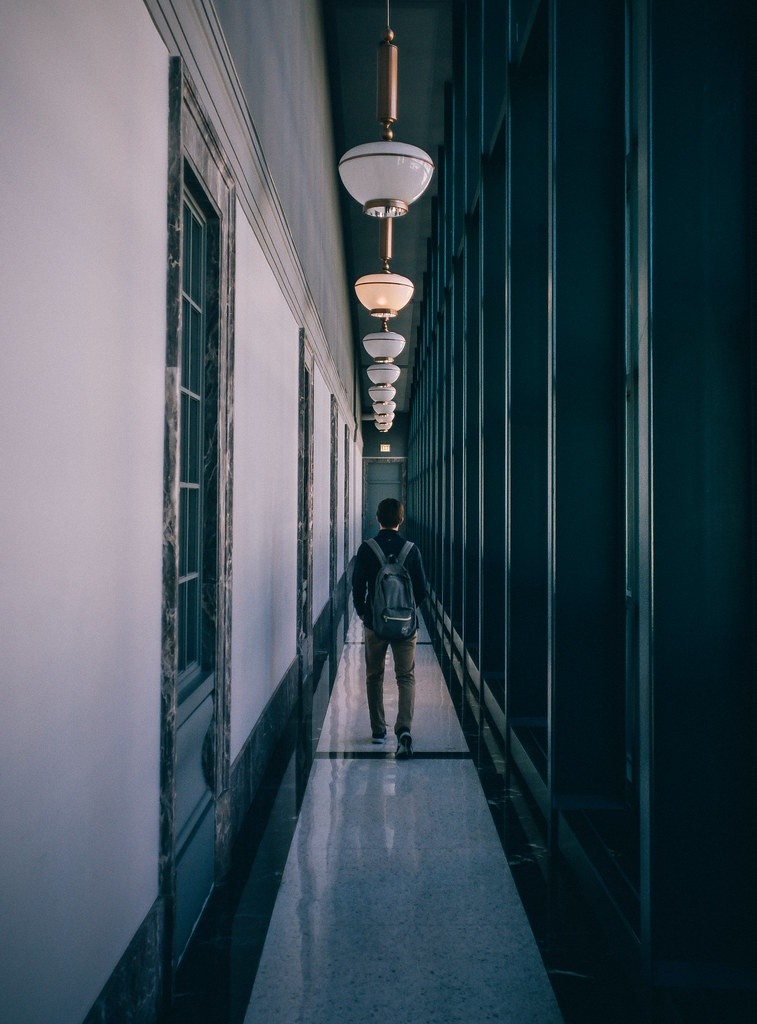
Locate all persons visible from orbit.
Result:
[352,498,428,759]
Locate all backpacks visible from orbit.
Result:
[363,537,419,643]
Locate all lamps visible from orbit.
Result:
[367,363,401,387]
[354,218,414,320]
[374,421,393,432]
[362,316,406,363]
[372,402,396,416]
[369,385,397,404]
[337,1,435,218]
[374,412,395,425]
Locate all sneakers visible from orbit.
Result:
[395,727,413,758]
[371,728,388,744]
